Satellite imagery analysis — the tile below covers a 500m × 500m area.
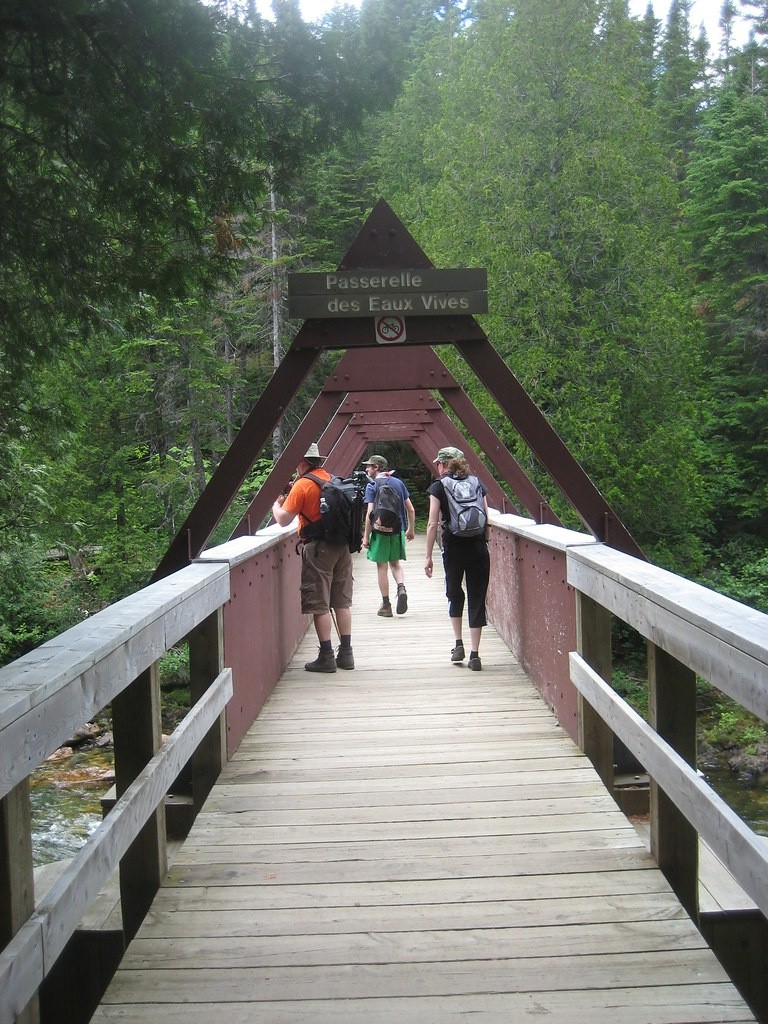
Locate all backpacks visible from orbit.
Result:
[441,475,488,536]
[299,473,365,553]
[369,478,403,535]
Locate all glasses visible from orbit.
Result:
[437,463,440,466]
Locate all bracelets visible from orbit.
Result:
[485,523,491,526]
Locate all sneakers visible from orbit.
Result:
[396,585,407,614]
[468,658,481,671]
[335,645,355,669]
[304,650,338,673]
[378,603,393,617]
[451,646,465,664]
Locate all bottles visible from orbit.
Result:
[320,498,329,513]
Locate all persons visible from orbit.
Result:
[271,443,354,673]
[362,455,415,617]
[424,446,491,671]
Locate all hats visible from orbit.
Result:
[362,455,389,468]
[303,442,329,459]
[433,447,465,465]
